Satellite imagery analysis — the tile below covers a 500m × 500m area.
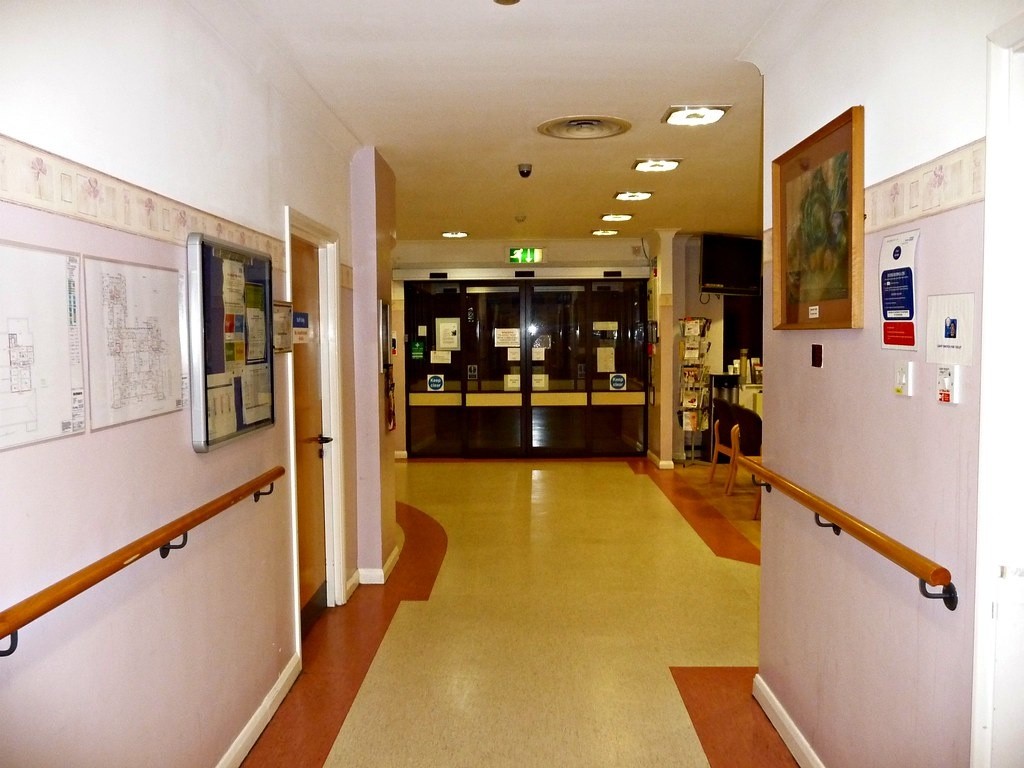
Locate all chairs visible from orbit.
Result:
[708,397,762,518]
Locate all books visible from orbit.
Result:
[680,319,709,431]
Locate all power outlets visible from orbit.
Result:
[895,362,912,395]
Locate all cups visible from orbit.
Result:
[728,365,733,374]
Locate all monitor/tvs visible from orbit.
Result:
[699,233,763,297]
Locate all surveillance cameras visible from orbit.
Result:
[519,164,532,177]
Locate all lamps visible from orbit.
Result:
[612,191,653,201]
[660,105,731,126]
[600,213,632,223]
[631,158,681,172]
[590,228,619,237]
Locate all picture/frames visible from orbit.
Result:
[272,300,294,353]
[379,299,391,374]
[771,105,864,329]
